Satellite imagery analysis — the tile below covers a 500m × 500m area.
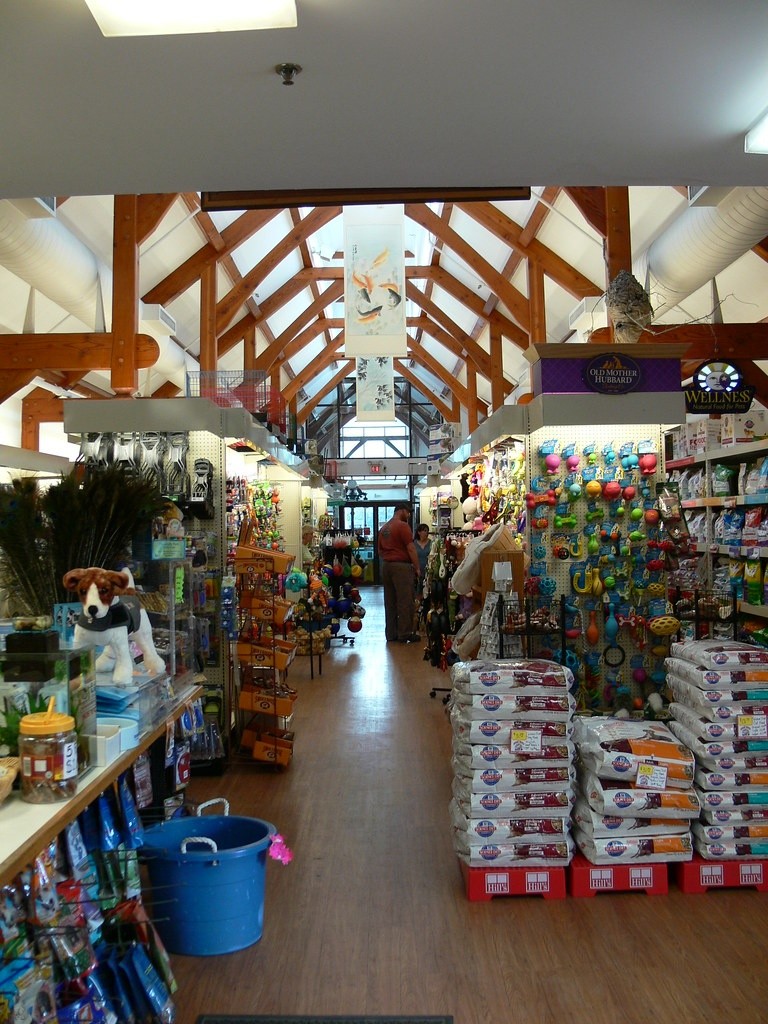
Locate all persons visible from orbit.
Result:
[413,522,432,592]
[377,503,422,642]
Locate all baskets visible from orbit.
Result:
[0,755,21,805]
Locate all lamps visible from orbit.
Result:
[341,476,367,502]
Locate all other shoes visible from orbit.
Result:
[403,634,421,642]
[387,635,398,641]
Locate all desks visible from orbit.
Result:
[0,685,204,889]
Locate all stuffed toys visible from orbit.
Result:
[434,534,474,631]
[308,575,329,607]
[454,462,484,522]
[62,567,166,684]
[285,567,307,592]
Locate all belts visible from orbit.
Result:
[386,560,410,563]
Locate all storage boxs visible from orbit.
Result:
[428,438,462,455]
[0,641,97,778]
[235,543,295,765]
[427,452,454,476]
[665,409,768,462]
[429,422,462,440]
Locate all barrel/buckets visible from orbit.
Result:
[141,815,277,957]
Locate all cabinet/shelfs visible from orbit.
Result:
[63,396,300,766]
[300,459,334,576]
[429,492,453,535]
[665,436,768,646]
[495,594,566,665]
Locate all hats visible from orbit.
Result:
[394,503,414,513]
[302,524,317,535]
